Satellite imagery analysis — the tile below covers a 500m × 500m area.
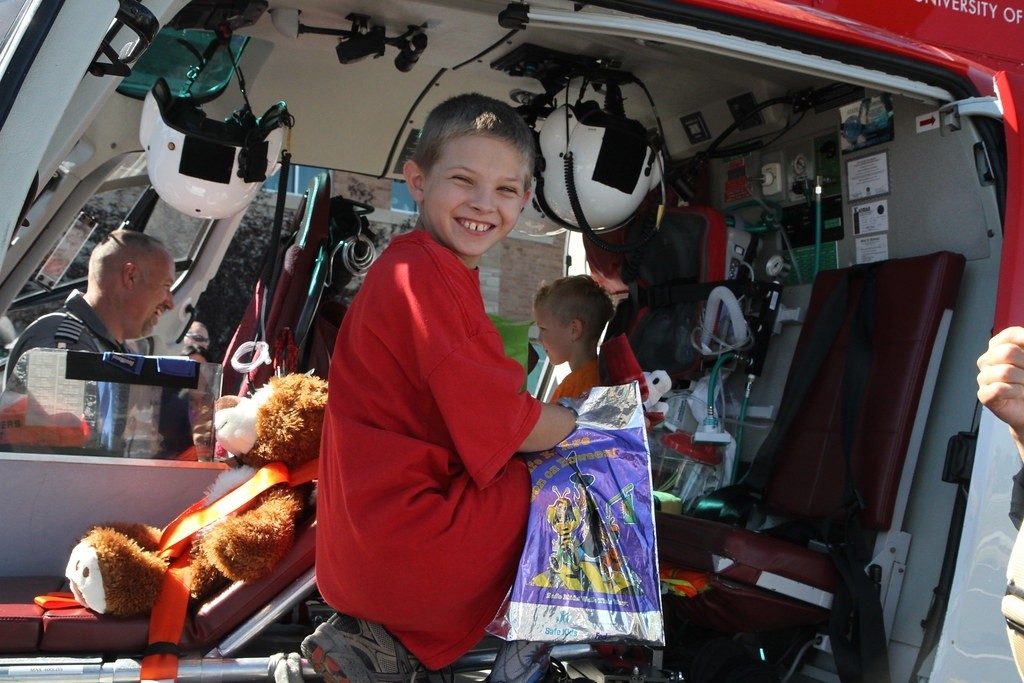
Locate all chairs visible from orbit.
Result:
[666,250,967,683]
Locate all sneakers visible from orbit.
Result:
[300,610,455,683]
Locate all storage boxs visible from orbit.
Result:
[15,347,224,459]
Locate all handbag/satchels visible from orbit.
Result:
[485,380,665,648]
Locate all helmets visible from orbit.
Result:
[139,77,291,220]
[537,102,664,229]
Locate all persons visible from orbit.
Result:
[5,227,175,390]
[534,276,614,406]
[977,323,1023,528]
[302,91,586,681]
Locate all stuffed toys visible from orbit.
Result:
[64,370,328,618]
